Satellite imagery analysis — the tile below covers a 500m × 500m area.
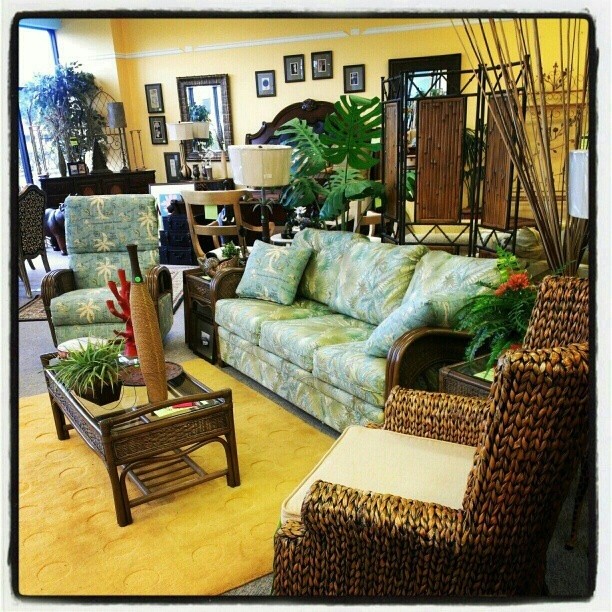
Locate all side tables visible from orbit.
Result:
[437,344,508,401]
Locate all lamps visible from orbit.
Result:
[107,102,131,174]
[228,143,291,246]
[191,122,209,151]
[168,119,195,167]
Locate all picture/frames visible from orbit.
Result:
[144,83,164,113]
[163,151,181,184]
[148,115,168,144]
[310,50,333,80]
[342,64,366,93]
[283,53,305,83]
[255,70,276,97]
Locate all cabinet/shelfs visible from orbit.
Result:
[378,53,531,259]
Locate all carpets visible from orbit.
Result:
[18,357,339,597]
[18,263,201,322]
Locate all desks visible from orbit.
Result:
[39,169,156,210]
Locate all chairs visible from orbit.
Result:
[17,183,51,299]
[181,189,274,264]
[272,274,592,601]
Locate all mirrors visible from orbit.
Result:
[176,72,233,162]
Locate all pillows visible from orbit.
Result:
[234,239,316,306]
[364,290,467,359]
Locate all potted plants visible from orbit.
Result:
[38,330,137,412]
[460,127,487,219]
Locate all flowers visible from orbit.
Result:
[493,272,537,297]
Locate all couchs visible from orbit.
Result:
[208,221,537,434]
[39,192,173,351]
